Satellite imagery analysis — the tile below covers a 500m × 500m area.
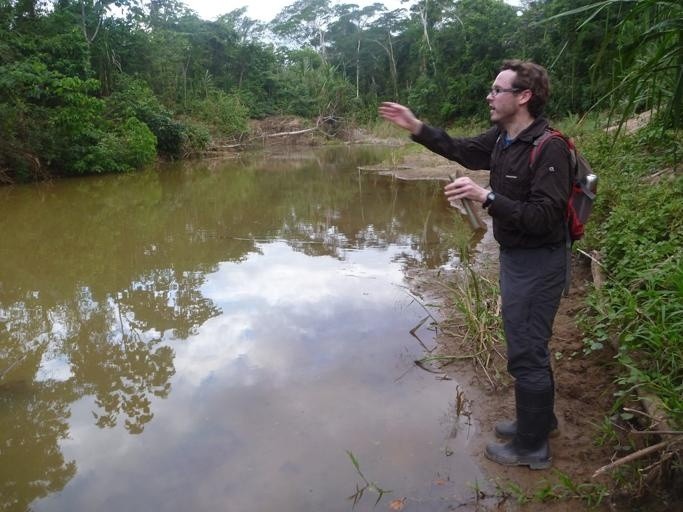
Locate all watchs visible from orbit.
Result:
[482,190,495,209]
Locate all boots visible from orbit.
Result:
[485,362,560,470]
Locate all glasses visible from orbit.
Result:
[491,87,520,95]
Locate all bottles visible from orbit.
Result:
[573,174,597,224]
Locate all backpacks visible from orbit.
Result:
[530,126,598,241]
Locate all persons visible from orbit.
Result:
[376,59,579,472]
